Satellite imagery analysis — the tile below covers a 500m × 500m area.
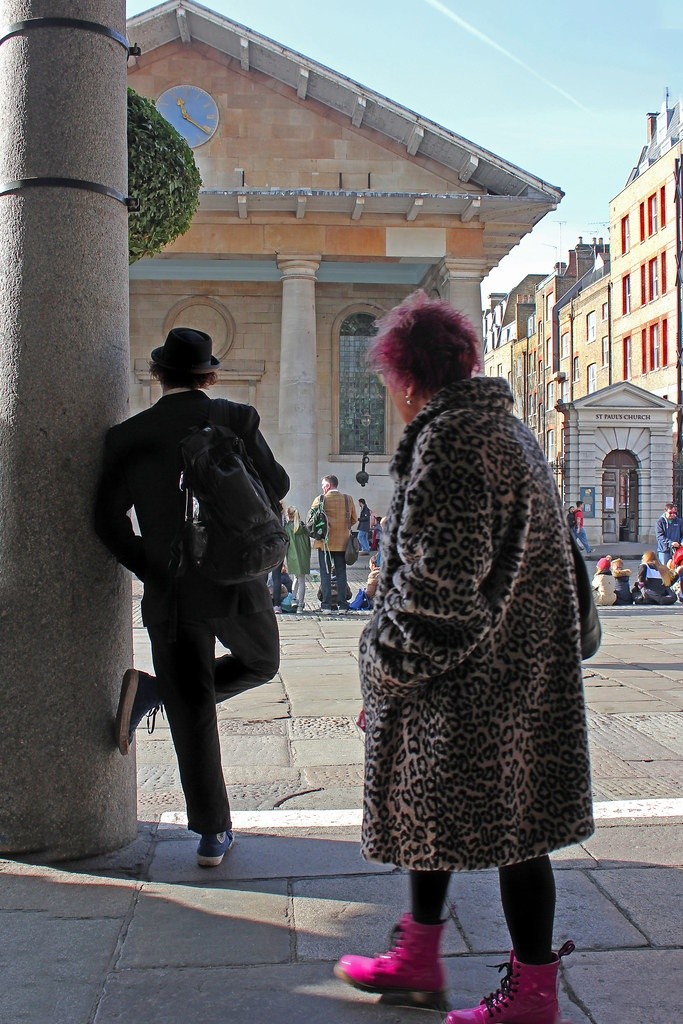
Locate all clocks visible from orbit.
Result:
[156,84,220,149]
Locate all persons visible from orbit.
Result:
[567,501,596,553]
[267,467,389,615]
[334,291,596,1024]
[591,504,683,606]
[92,328,291,865]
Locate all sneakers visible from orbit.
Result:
[114,668,165,755]
[196,829,236,866]
[296,607,304,614]
[291,600,297,607]
[274,606,283,614]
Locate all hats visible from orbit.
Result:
[151,327,221,373]
[596,555,612,571]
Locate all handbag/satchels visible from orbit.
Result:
[344,530,361,566]
[566,529,603,660]
[305,494,328,540]
[317,578,353,604]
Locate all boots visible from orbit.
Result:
[442,940,575,1024]
[333,914,450,994]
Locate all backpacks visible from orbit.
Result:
[150,398,293,588]
[567,509,581,528]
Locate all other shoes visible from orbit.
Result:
[580,548,585,552]
[338,601,351,605]
[587,548,596,554]
[360,551,370,556]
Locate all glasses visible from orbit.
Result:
[666,510,677,515]
